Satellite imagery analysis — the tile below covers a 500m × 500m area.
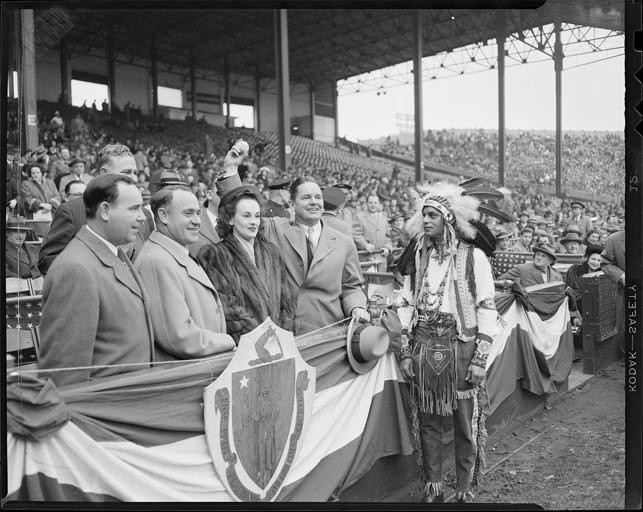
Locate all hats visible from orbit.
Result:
[346,318,389,375]
[559,224,603,255]
[322,184,352,207]
[5,216,32,230]
[268,179,290,190]
[153,169,187,185]
[217,185,261,212]
[569,202,584,209]
[68,158,86,166]
[531,243,556,260]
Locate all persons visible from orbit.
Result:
[7,86,624,313]
[40,173,157,386]
[396,174,514,504]
[598,228,626,357]
[218,138,370,336]
[199,186,296,345]
[135,188,238,358]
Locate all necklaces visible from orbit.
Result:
[420,247,452,325]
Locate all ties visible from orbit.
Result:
[308,228,317,256]
